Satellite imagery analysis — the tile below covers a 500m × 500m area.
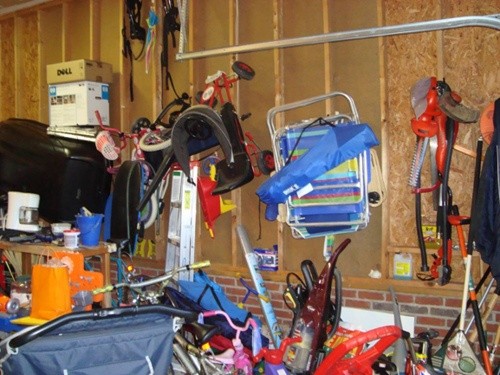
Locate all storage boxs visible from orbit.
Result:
[46,58,113,128]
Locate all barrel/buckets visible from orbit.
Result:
[74,214,104,246]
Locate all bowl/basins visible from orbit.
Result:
[50,222,71,235]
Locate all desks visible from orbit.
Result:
[0,242,117,308]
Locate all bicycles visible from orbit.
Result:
[202,309,259,374]
[90,260,233,375]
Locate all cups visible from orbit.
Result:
[63,230,80,248]
